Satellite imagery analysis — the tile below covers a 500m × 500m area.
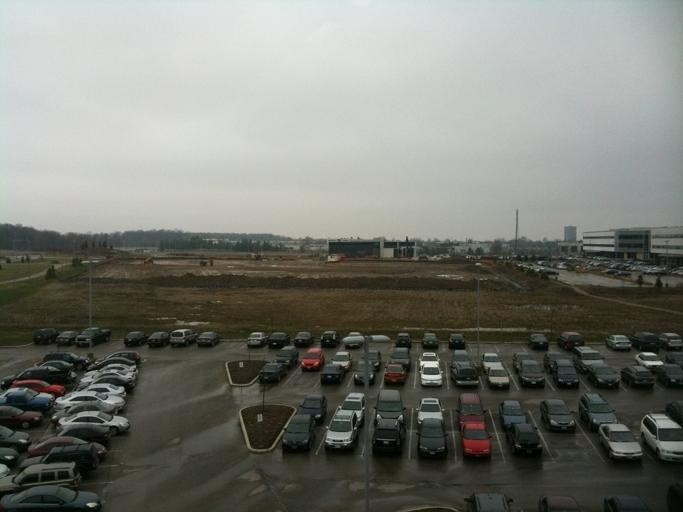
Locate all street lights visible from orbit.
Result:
[474,260,482,362]
[81,258,101,351]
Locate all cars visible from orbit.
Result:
[0,349,144,512]
[400,476,683,512]
[416,253,450,260]
[514,257,683,279]
[30,326,221,349]
